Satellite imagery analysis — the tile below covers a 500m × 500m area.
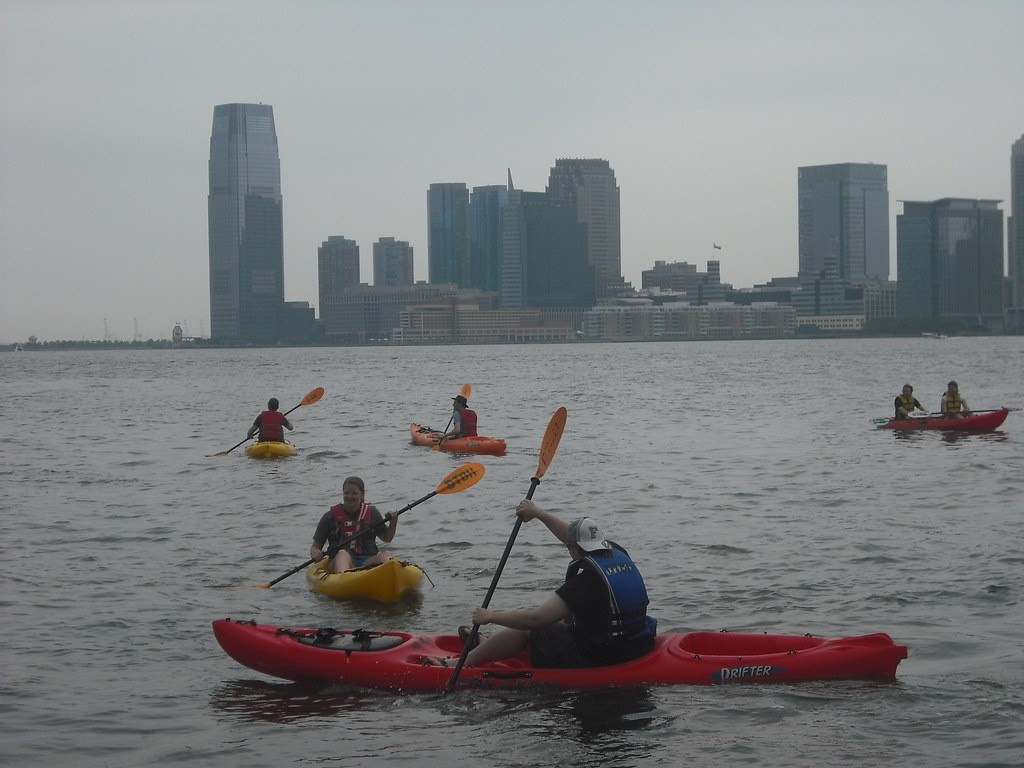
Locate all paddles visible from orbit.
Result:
[213,387,324,455]
[442,407,567,705]
[252,461,485,589]
[432,383,471,452]
[907,407,1024,418]
[867,417,907,424]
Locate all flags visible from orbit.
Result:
[714,244,721,250]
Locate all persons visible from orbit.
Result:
[308,475,399,575]
[893,384,930,420]
[426,498,659,674]
[939,381,975,419]
[436,395,478,442]
[244,397,294,443]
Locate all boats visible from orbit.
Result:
[885,406,1009,434]
[210,612,910,690]
[409,422,506,456]
[244,439,254,457]
[307,552,428,601]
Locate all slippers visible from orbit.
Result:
[458,626,487,650]
[423,657,449,666]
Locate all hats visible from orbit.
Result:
[451,395,469,408]
[568,517,612,552]
